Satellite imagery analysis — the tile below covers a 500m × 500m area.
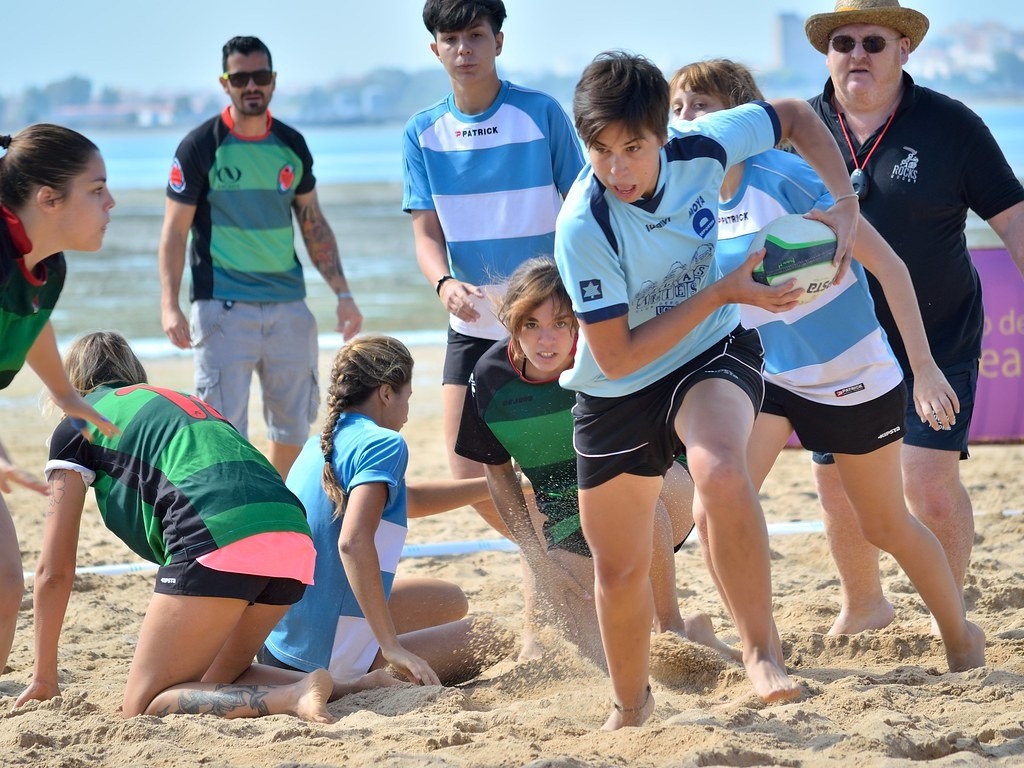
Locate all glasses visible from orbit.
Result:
[223,69,273,88]
[829,37,899,54]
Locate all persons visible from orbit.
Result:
[11,331,407,725]
[156,36,362,480]
[254,0,1024,767]
[0,123,123,673]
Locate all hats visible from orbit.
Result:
[806,1,930,55]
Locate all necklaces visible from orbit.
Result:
[521,354,526,377]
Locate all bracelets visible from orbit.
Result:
[436,275,454,297]
[338,292,352,298]
[835,193,859,204]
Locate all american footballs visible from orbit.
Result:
[745,213,841,304]
